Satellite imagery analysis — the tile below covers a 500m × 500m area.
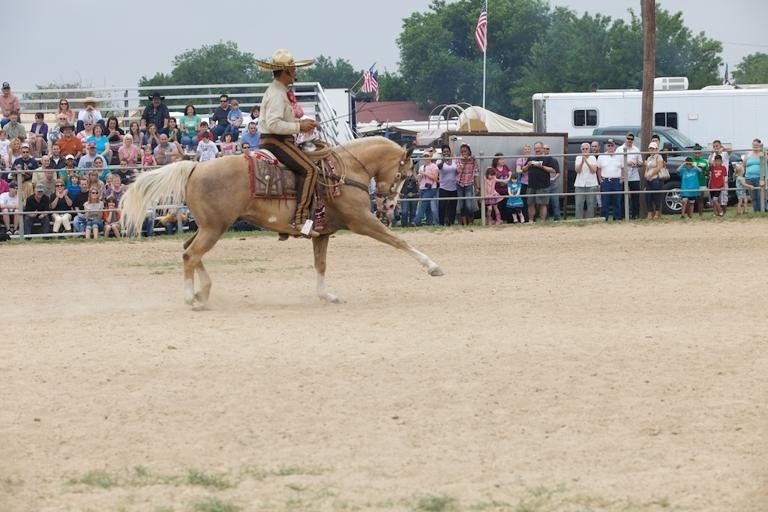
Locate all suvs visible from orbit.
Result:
[536,134,713,214]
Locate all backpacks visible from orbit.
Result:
[403,175,418,194]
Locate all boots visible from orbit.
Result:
[462,211,524,227]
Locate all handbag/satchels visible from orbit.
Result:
[656,155,671,180]
[515,173,523,187]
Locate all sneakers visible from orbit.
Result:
[529,215,546,224]
[5,230,19,235]
[712,210,728,217]
[401,221,455,227]
[289,221,320,237]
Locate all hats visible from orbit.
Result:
[34,184,46,192]
[255,48,316,71]
[1,82,10,90]
[608,138,616,145]
[87,142,97,149]
[9,181,18,189]
[149,93,165,101]
[423,151,432,157]
[60,122,75,133]
[66,154,75,160]
[693,144,701,150]
[648,142,658,148]
[81,96,102,103]
[627,131,635,137]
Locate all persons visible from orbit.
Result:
[1,82,266,242]
[368,131,766,229]
[260,68,322,241]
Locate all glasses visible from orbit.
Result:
[627,138,634,140]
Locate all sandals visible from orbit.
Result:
[646,211,661,220]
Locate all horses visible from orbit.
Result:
[116,135,444,309]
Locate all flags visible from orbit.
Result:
[363,68,372,93]
[474,1,487,53]
[367,71,379,102]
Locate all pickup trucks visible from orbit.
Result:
[591,126,743,205]
